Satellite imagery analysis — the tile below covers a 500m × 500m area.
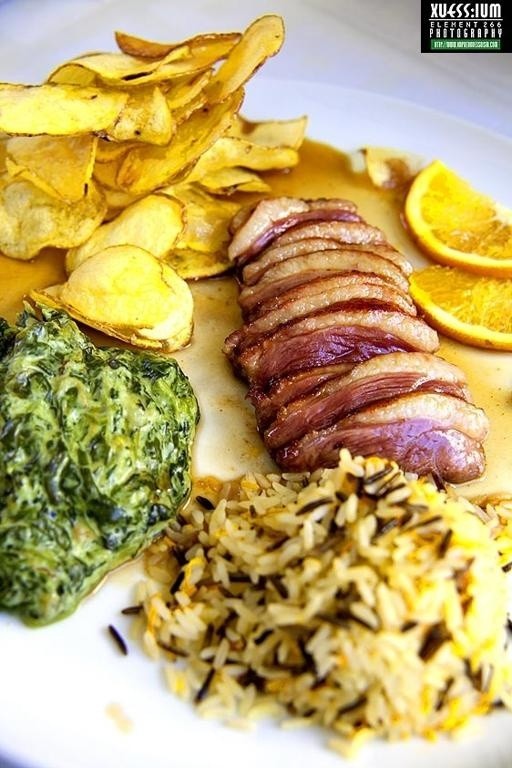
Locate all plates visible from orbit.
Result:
[0,65,511,766]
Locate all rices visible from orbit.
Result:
[105,446,512,759]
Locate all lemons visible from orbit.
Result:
[407,265,512,351]
[403,158,512,276]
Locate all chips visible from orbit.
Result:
[0,13,307,355]
[359,147,424,189]
